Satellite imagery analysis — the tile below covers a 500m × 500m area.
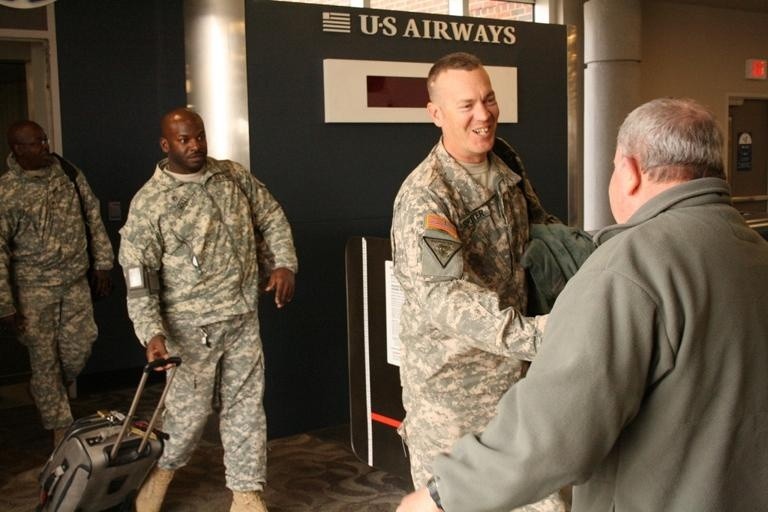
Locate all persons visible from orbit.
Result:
[118,107,300,512]
[0,119,115,446]
[390,52,572,512]
[391,97,767,512]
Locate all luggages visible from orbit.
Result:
[37,356,181,512]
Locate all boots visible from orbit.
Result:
[230,490,268,511]
[134,470,174,512]
